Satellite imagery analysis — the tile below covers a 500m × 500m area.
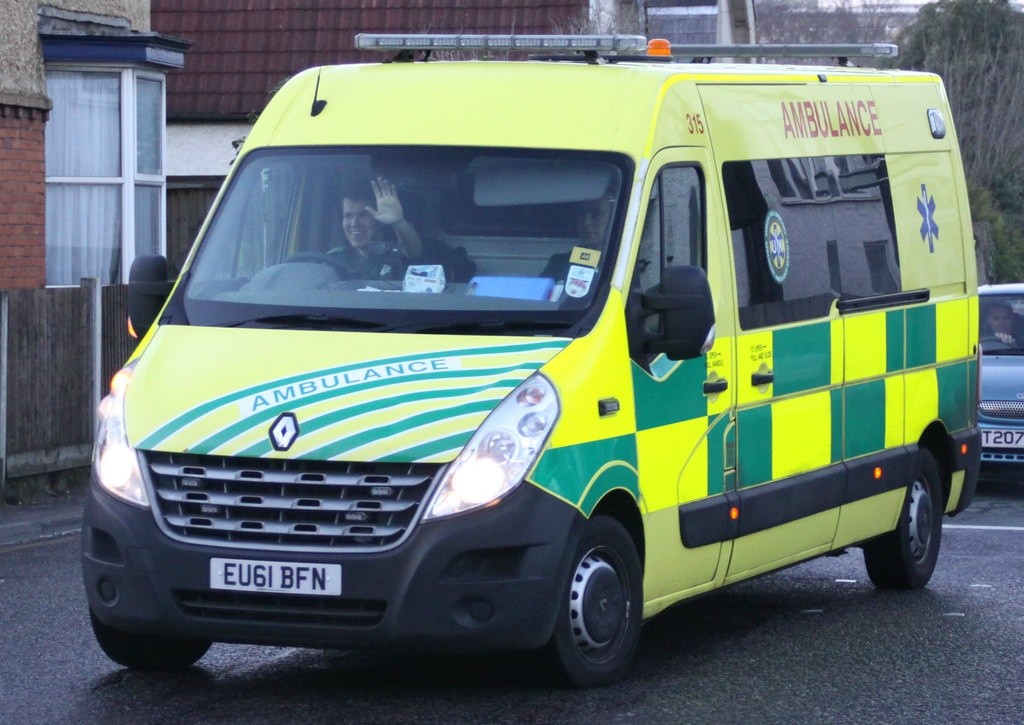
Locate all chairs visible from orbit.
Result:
[382,188,445,265]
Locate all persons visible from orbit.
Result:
[538,185,614,291]
[317,173,423,279]
[980,301,1020,349]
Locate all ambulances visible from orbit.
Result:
[78,29,981,692]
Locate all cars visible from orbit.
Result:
[978,283,1024,465]
